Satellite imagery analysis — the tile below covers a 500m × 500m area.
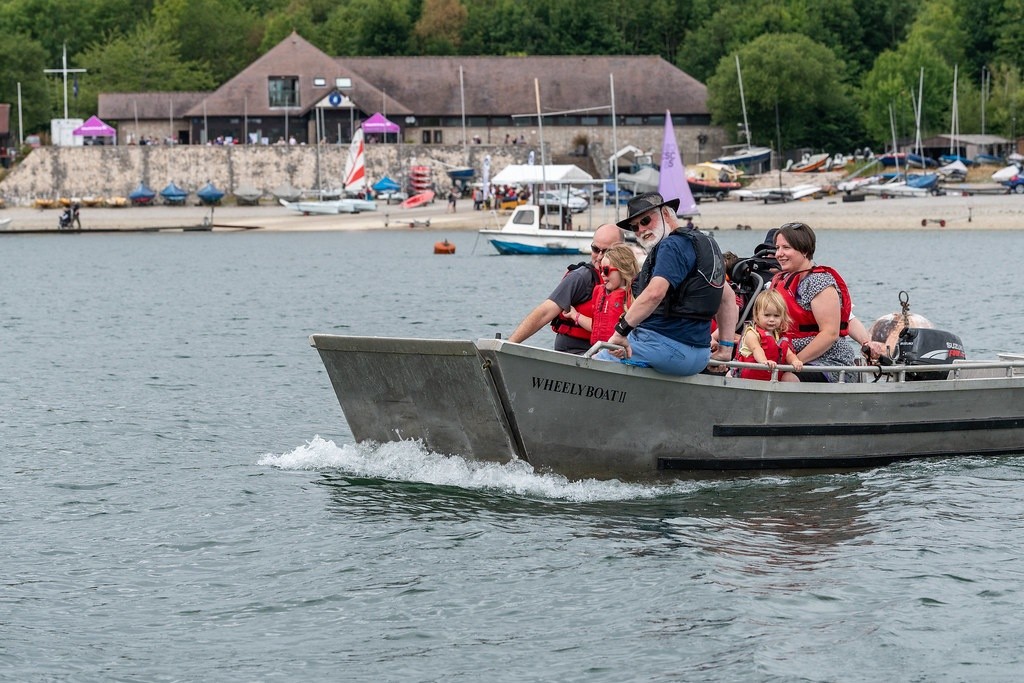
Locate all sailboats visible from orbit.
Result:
[934,90,969,182]
[233,97,263,202]
[973,65,1006,164]
[938,62,972,166]
[711,55,773,166]
[198,98,224,205]
[127,100,156,203]
[279,122,378,215]
[158,94,188,201]
[273,93,301,201]
[906,67,938,168]
[837,87,944,197]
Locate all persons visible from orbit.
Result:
[472,184,516,210]
[470,136,481,144]
[508,224,624,356]
[592,192,736,376]
[562,247,639,353]
[704,226,884,377]
[764,222,859,383]
[278,136,285,144]
[725,289,803,381]
[206,134,239,146]
[447,186,456,212]
[516,134,527,145]
[140,136,159,145]
[289,135,297,145]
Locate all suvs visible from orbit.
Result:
[525,190,588,213]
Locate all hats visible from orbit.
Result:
[754,228,780,258]
[617,191,680,231]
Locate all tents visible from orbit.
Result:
[71,113,116,146]
[361,112,400,143]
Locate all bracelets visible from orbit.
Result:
[719,340,735,347]
[575,312,580,326]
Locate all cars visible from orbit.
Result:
[1001,168,1024,194]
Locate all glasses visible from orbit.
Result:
[599,265,622,277]
[780,223,812,241]
[591,242,613,254]
[629,209,663,232]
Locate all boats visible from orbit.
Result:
[1005,152,1024,166]
[446,164,474,180]
[478,204,638,255]
[106,196,129,208]
[686,178,742,191]
[309,333,1024,486]
[373,177,402,195]
[35,197,54,207]
[60,197,80,207]
[790,147,876,173]
[82,195,102,207]
